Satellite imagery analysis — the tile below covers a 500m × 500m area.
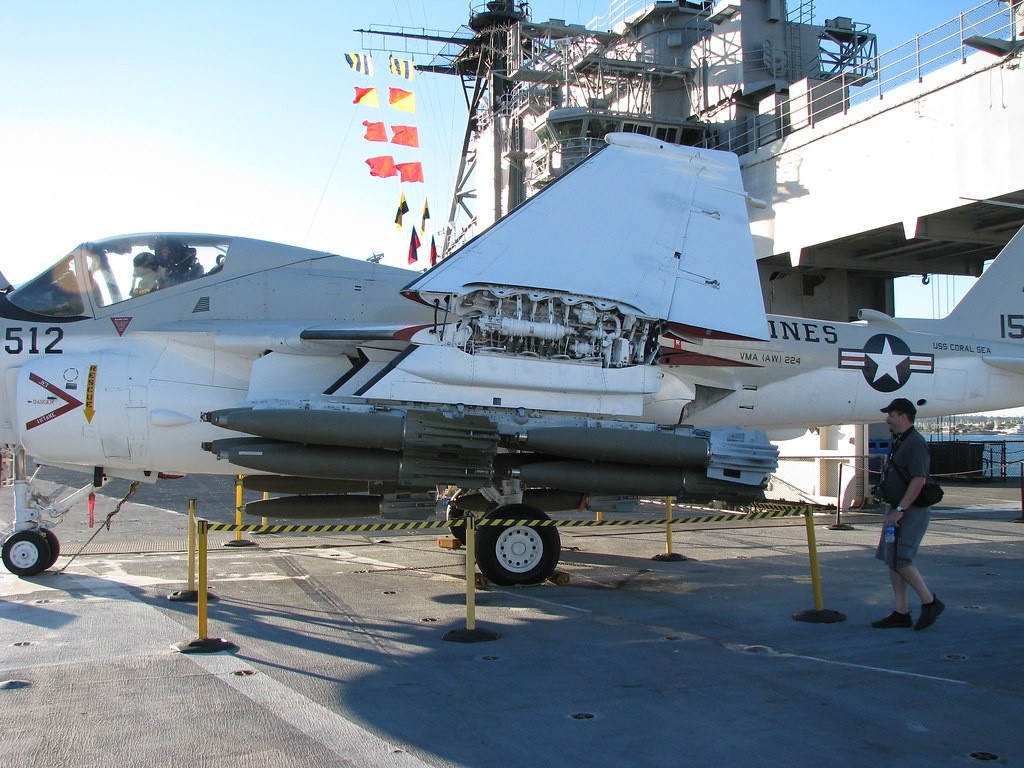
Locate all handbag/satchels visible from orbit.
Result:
[912,483,944,509]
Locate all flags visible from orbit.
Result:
[397,162,424,183]
[354,87,379,108]
[366,156,397,178]
[363,121,388,142]
[430,237,437,266]
[345,54,373,76]
[396,193,409,228]
[391,125,419,147]
[422,201,431,235]
[389,57,413,80]
[408,227,420,264]
[389,87,415,113]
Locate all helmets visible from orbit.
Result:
[133,252,158,272]
[150,239,186,268]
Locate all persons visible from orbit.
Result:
[130,252,158,295]
[869,398,944,630]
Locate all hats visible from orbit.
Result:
[880,398,917,415]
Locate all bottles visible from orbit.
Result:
[884,525,895,543]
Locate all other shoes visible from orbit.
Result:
[915,593,944,630]
[872,611,913,628]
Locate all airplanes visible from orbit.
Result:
[0,132,1024,581]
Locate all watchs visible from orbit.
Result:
[897,506,905,512]
